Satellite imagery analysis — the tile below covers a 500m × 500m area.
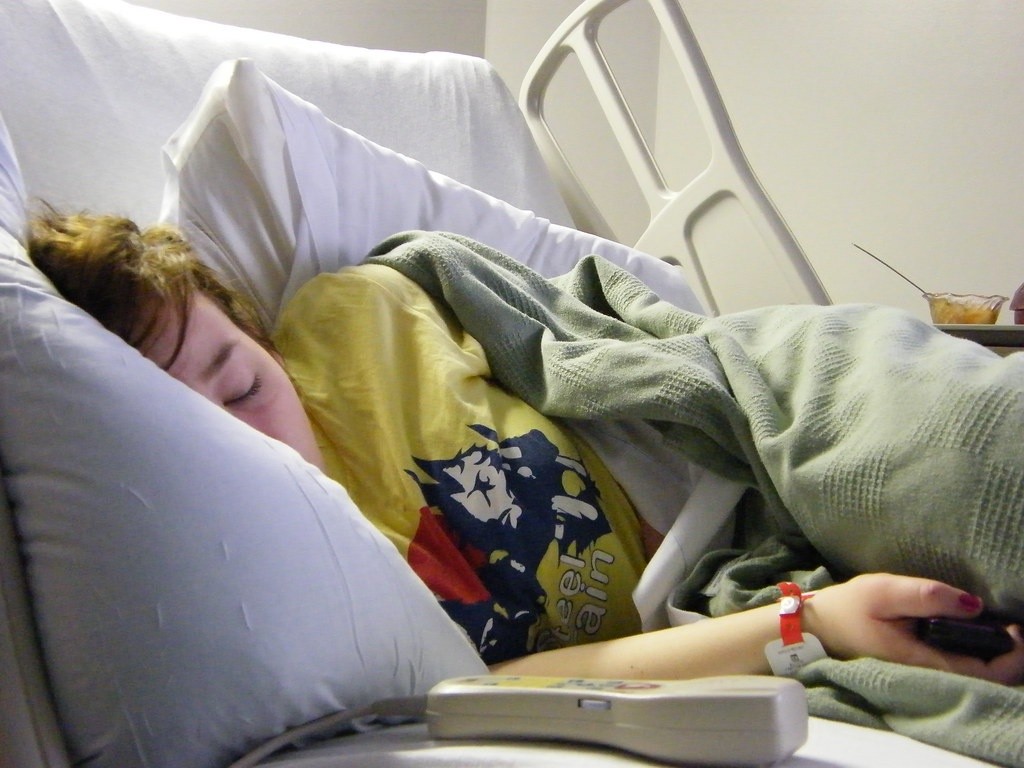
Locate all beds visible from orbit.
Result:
[0,0,1013,768]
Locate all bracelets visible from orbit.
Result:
[766,582,826,677]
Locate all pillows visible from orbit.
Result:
[0,115,491,768]
[155,52,744,561]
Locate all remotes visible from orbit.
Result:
[424,676,809,767]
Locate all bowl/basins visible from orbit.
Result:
[923,293,1011,326]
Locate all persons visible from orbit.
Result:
[34,217,1024,686]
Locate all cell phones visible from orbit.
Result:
[916,618,1013,658]
